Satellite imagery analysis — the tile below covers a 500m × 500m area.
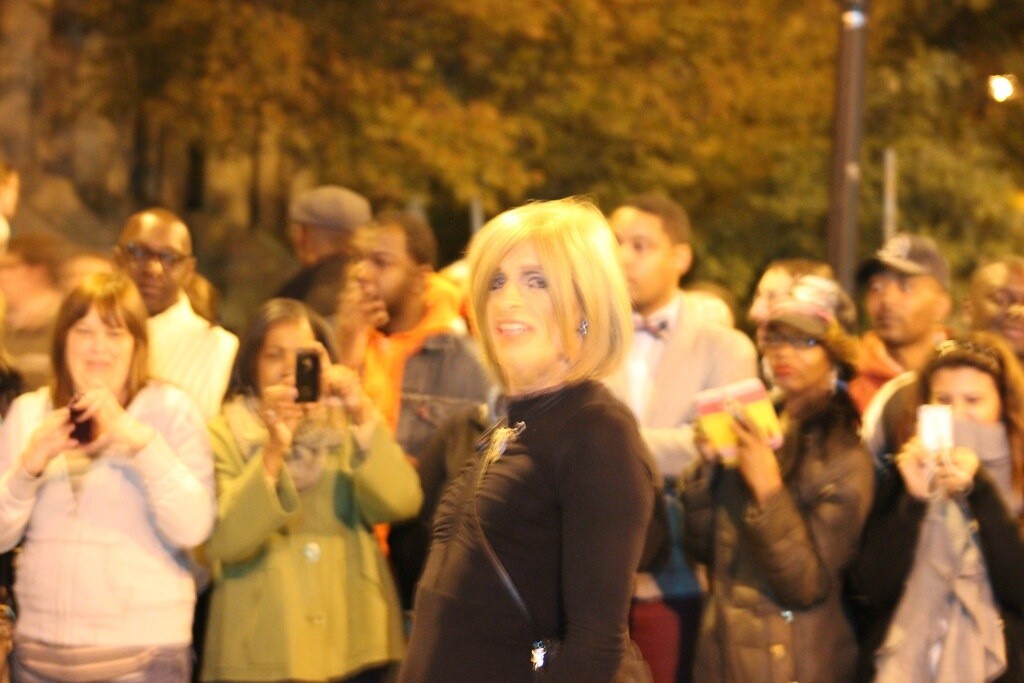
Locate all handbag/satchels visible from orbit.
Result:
[529,628,651,683]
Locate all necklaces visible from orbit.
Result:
[489,386,573,462]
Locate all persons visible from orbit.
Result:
[399,195,656,683]
[0,151,1024,683]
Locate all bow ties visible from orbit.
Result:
[632,317,666,339]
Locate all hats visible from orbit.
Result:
[764,276,857,344]
[291,188,372,233]
[855,231,949,283]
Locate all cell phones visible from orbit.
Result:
[294,353,320,403]
[920,403,952,459]
[70,406,92,445]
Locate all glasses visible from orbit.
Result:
[760,330,819,350]
[120,245,193,271]
